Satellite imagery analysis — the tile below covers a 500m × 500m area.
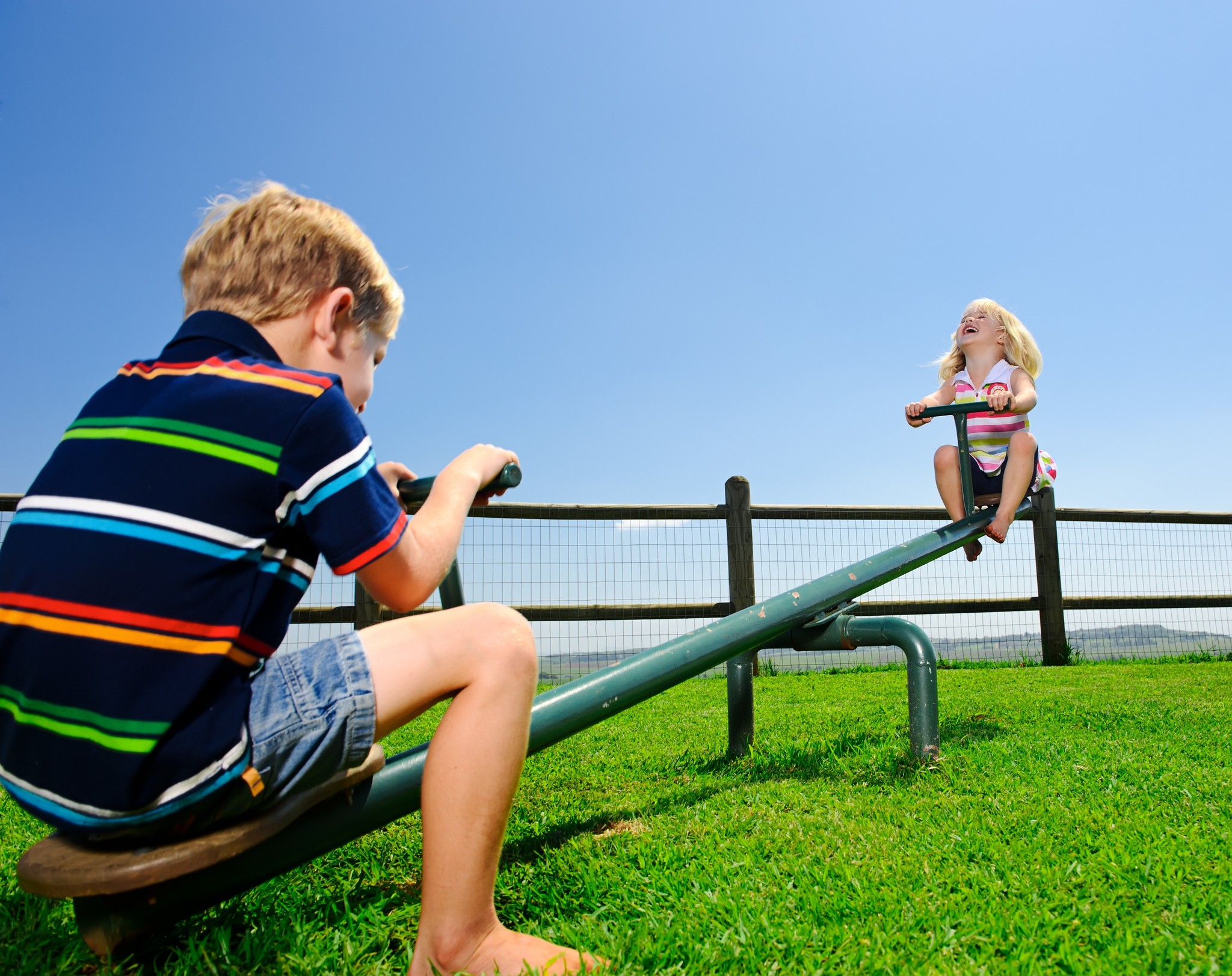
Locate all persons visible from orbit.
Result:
[0,179,611,976]
[905,298,1057,562]
